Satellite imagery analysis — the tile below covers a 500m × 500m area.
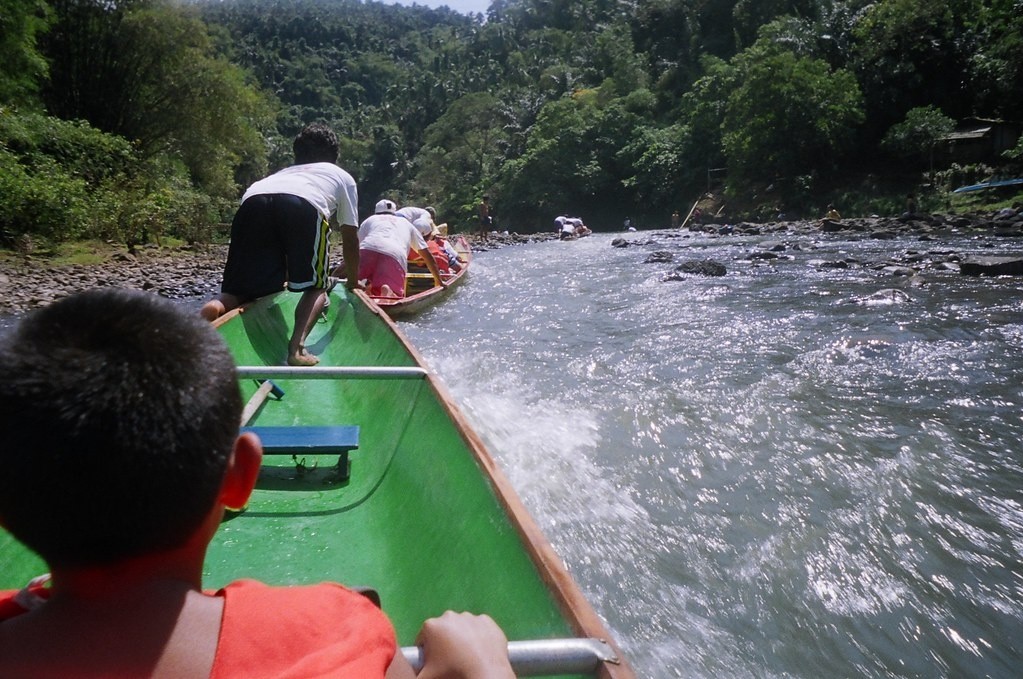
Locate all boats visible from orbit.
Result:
[574,226,592,238]
[361,234,472,317]
[0,278,634,678]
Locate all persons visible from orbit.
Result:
[992,202,1022,217]
[561,224,574,240]
[395,207,437,231]
[554,214,568,236]
[333,201,449,303]
[479,193,490,243]
[817,204,840,227]
[566,217,585,230]
[903,193,917,218]
[672,210,679,229]
[430,240,468,263]
[408,219,462,272]
[0,285,516,679]
[626,226,637,232]
[199,122,360,366]
[694,208,701,217]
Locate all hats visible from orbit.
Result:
[375,199,396,214]
[413,218,432,236]
